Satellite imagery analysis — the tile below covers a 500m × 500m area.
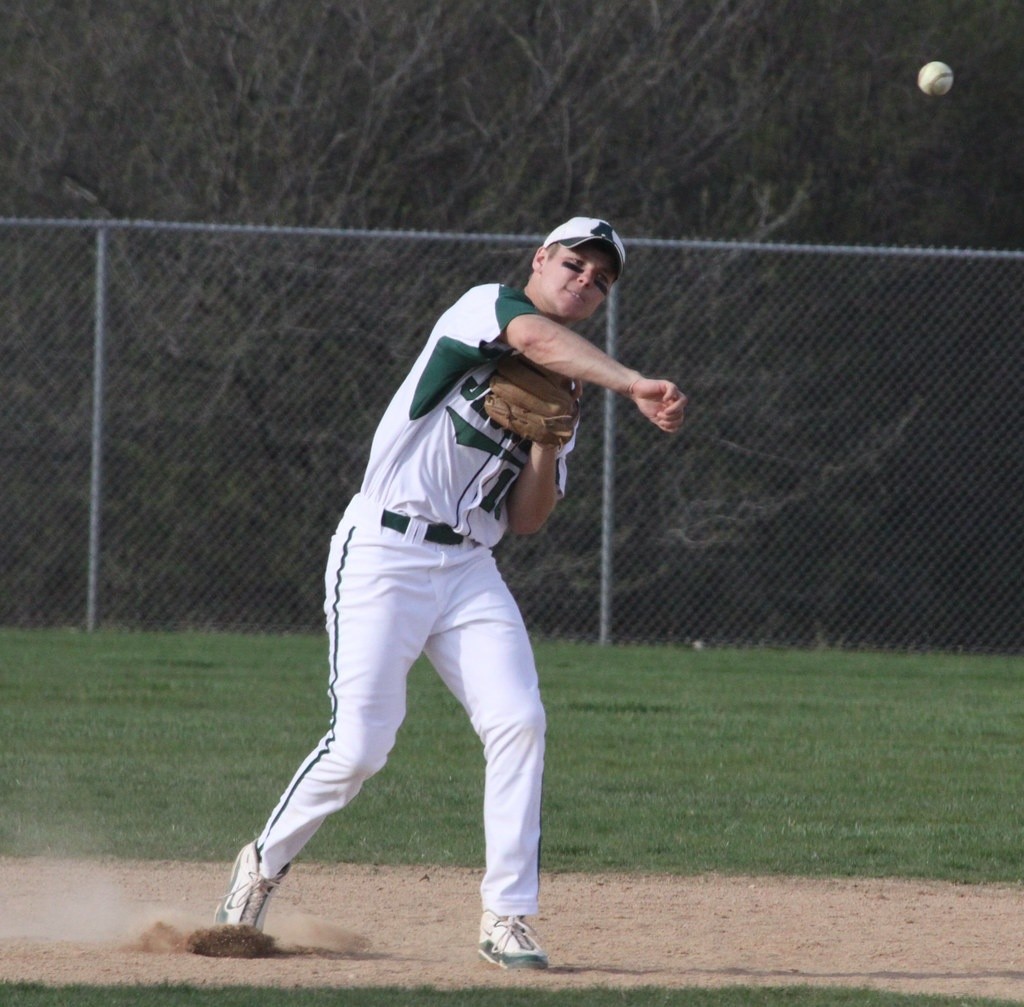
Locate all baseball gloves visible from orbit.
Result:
[483,353,582,449]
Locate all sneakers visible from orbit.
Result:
[478,910,548,971]
[212,836,292,932]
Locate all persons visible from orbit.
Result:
[215,215,689,971]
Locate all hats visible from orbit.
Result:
[543,217,626,285]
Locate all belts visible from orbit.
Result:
[381,510,463,545]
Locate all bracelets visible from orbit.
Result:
[533,445,561,453]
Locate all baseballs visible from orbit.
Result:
[917,59,956,97]
